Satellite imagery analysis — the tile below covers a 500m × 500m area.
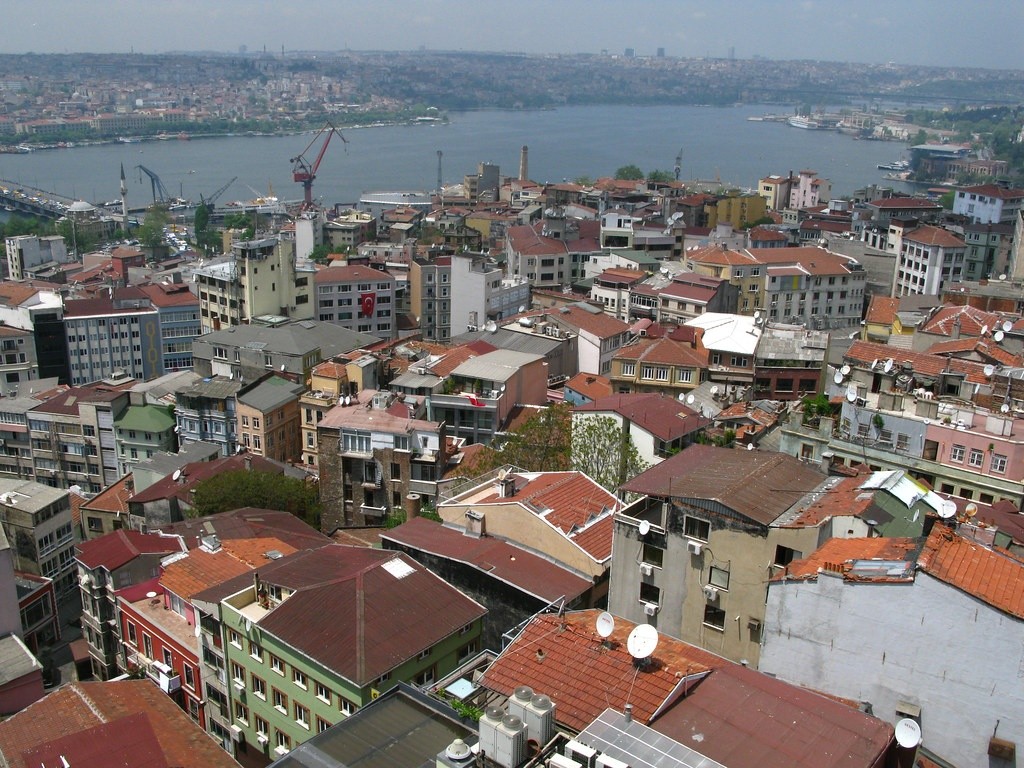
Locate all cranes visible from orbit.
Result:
[190,174,239,209]
[289,118,351,208]
[131,163,170,209]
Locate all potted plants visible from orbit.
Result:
[412,447,421,458]
[872,414,885,432]
[447,376,466,395]
[472,379,482,397]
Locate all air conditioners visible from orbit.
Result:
[203,301,207,306]
[595,752,629,768]
[639,563,653,577]
[234,684,245,696]
[643,603,657,617]
[257,737,266,747]
[553,329,560,338]
[703,585,719,601]
[549,753,582,768]
[417,368,425,375]
[133,303,140,309]
[545,327,553,336]
[565,739,597,768]
[687,541,702,556]
[274,747,286,759]
[262,255,267,260]
[218,288,222,293]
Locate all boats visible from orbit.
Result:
[788,115,818,130]
[836,121,865,135]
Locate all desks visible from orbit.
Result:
[146,591,160,610]
